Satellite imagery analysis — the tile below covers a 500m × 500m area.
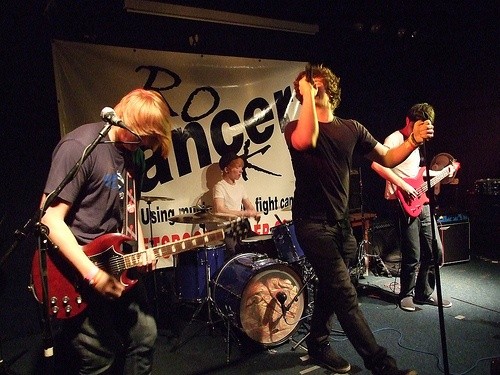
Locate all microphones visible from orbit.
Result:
[305,64,313,83]
[277,292,287,318]
[100,107,126,129]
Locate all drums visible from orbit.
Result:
[173,243,226,307]
[240,233,274,256]
[211,251,310,348]
[270,220,307,264]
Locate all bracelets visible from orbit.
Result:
[83,265,99,283]
[406,136,419,149]
[410,132,424,147]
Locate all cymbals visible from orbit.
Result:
[167,212,235,224]
[281,205,292,211]
[139,195,175,203]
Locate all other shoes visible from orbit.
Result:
[413,292,453,307]
[308,341,351,373]
[400,296,416,310]
[388,369,419,375]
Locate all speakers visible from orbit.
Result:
[434,221,471,265]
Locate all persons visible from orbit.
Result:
[370,103,456,311]
[213,154,261,237]
[284,66,434,375]
[39,89,169,375]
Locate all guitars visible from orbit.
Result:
[396,160,460,218]
[31,216,255,322]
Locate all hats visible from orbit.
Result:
[218,152,240,170]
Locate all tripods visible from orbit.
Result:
[168,222,242,353]
[349,166,392,279]
